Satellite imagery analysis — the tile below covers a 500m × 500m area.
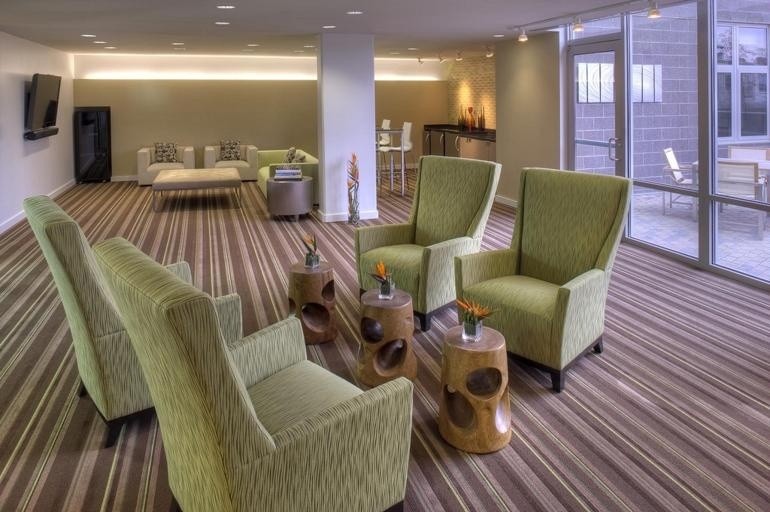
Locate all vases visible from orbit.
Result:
[304,252,320,269]
[461,321,483,343]
[379,281,395,300]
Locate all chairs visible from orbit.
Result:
[360,155,502,331]
[89,233,416,512]
[204,143,258,181]
[136,144,195,186]
[374,119,391,180]
[377,121,417,190]
[453,165,635,393]
[656,142,769,238]
[22,194,245,449]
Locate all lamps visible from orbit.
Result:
[505,1,666,42]
[415,45,495,63]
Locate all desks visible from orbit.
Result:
[267,174,315,222]
[357,288,417,387]
[150,168,242,211]
[288,262,338,348]
[441,325,513,454]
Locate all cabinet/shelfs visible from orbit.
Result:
[425,128,496,162]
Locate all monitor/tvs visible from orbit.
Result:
[25,73,62,131]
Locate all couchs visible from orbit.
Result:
[257,148,320,204]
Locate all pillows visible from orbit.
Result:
[293,155,307,163]
[153,140,177,162]
[281,146,296,162]
[219,138,241,160]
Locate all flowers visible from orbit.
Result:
[457,295,495,336]
[298,233,322,267]
[370,262,399,296]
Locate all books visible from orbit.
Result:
[274,167,302,180]
